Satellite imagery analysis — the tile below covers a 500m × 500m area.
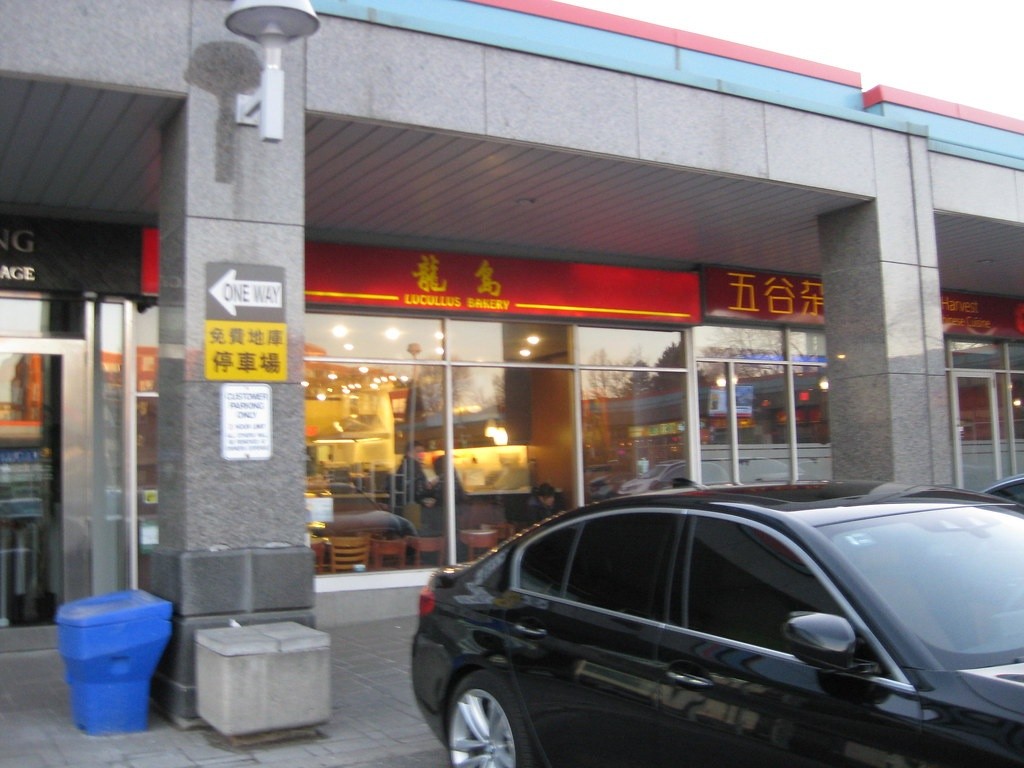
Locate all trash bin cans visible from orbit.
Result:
[52,589,174,736]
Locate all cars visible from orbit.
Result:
[723,458,809,482]
[407,476,1024,768]
[304,478,417,570]
[618,456,732,495]
[979,475,1024,512]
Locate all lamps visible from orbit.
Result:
[484,419,509,446]
[225,0,320,143]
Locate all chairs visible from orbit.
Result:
[479,523,514,541]
[457,530,498,560]
[312,531,447,575]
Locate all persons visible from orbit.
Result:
[525,482,563,527]
[394,441,468,564]
